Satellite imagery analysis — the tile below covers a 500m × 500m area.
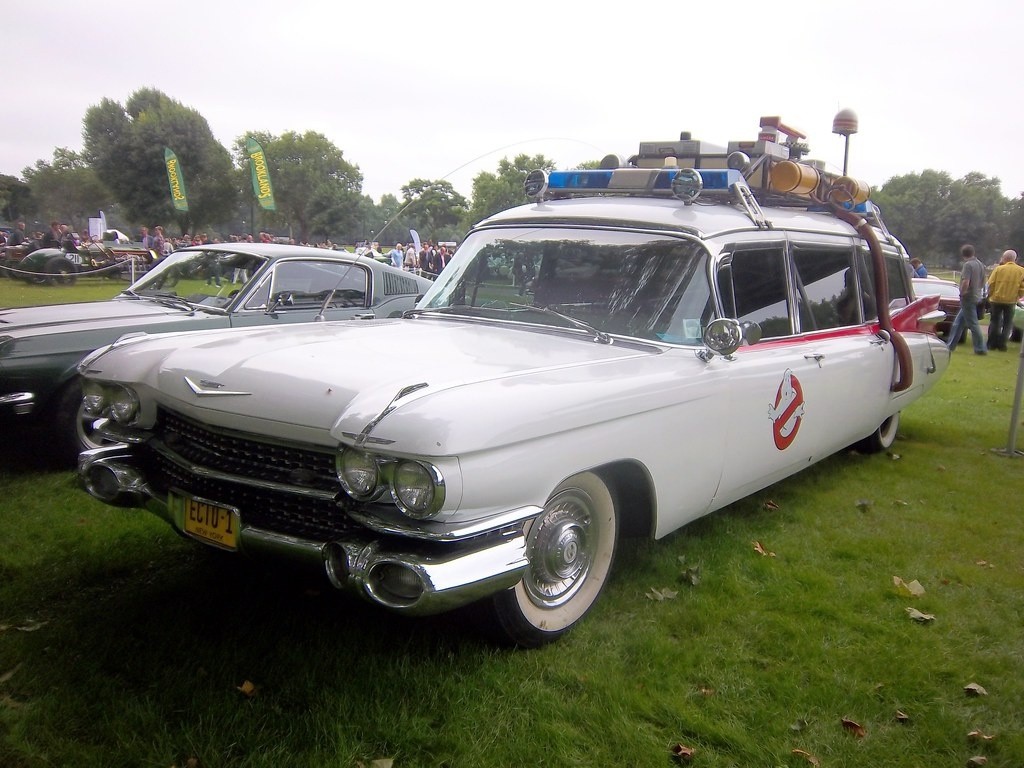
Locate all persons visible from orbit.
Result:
[947,245,988,354]
[985,250,1024,352]
[1,222,89,257]
[140,225,295,287]
[910,258,927,278]
[391,239,454,280]
[299,238,382,259]
[486,247,543,297]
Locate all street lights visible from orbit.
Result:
[384,219,388,245]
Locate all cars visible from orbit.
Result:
[66,105,952,649]
[1,235,509,466]
[0,223,159,286]
[910,277,969,344]
[988,298,1024,342]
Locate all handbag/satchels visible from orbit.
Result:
[977,301,984,320]
